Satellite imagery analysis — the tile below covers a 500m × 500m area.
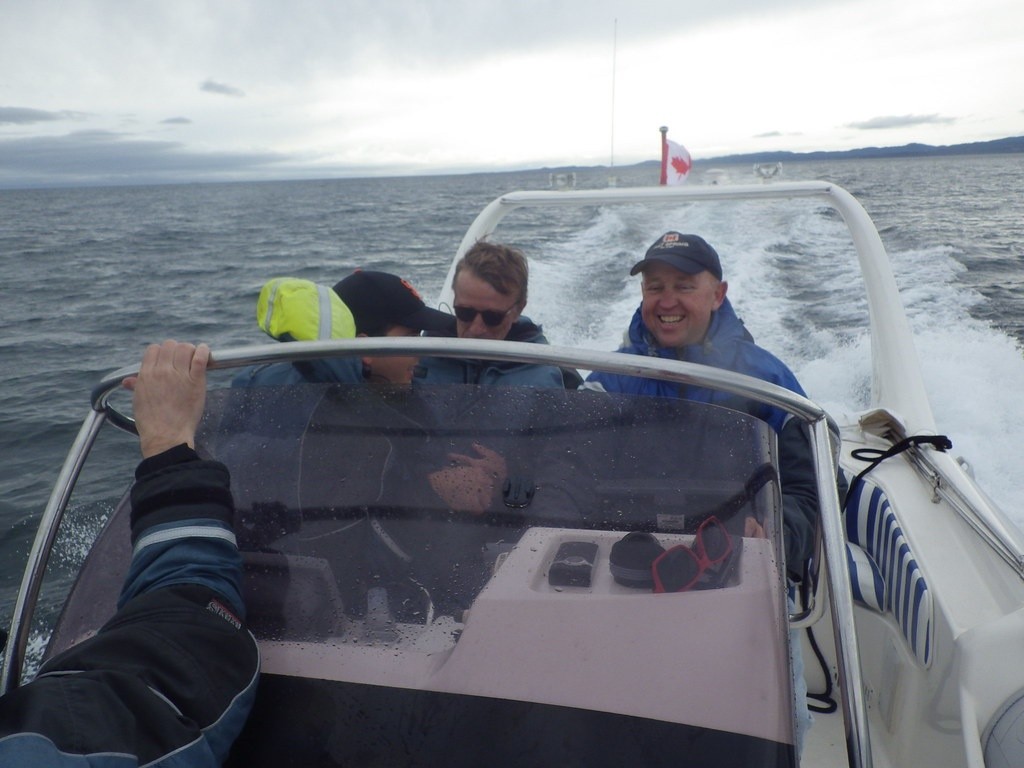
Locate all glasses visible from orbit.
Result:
[453,302,519,326]
[651,515,734,595]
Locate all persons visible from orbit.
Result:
[208,268,453,621]
[402,239,586,622]
[574,231,819,587]
[0,339,261,768]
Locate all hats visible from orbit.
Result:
[333,269,456,334]
[630,231,723,281]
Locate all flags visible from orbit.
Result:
[659,138,692,185]
